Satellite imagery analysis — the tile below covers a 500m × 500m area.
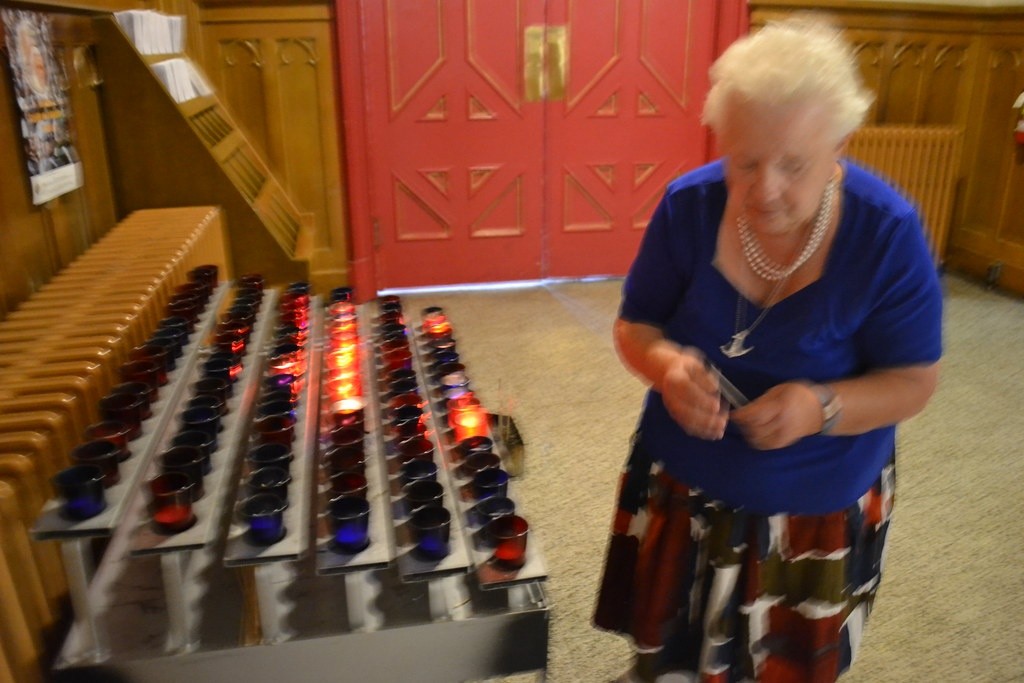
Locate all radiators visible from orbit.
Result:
[0,204,235,683]
[840,125,963,278]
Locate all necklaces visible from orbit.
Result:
[719,169,834,358]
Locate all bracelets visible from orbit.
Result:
[815,385,842,435]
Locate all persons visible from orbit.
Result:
[589,17,941,682]
